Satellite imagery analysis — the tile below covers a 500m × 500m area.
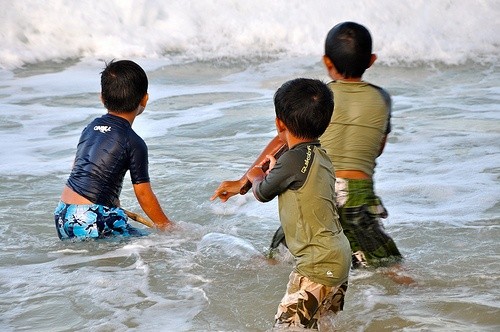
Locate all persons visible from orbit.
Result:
[55,59,178,244]
[247,79,352,332]
[210,22,418,290]
